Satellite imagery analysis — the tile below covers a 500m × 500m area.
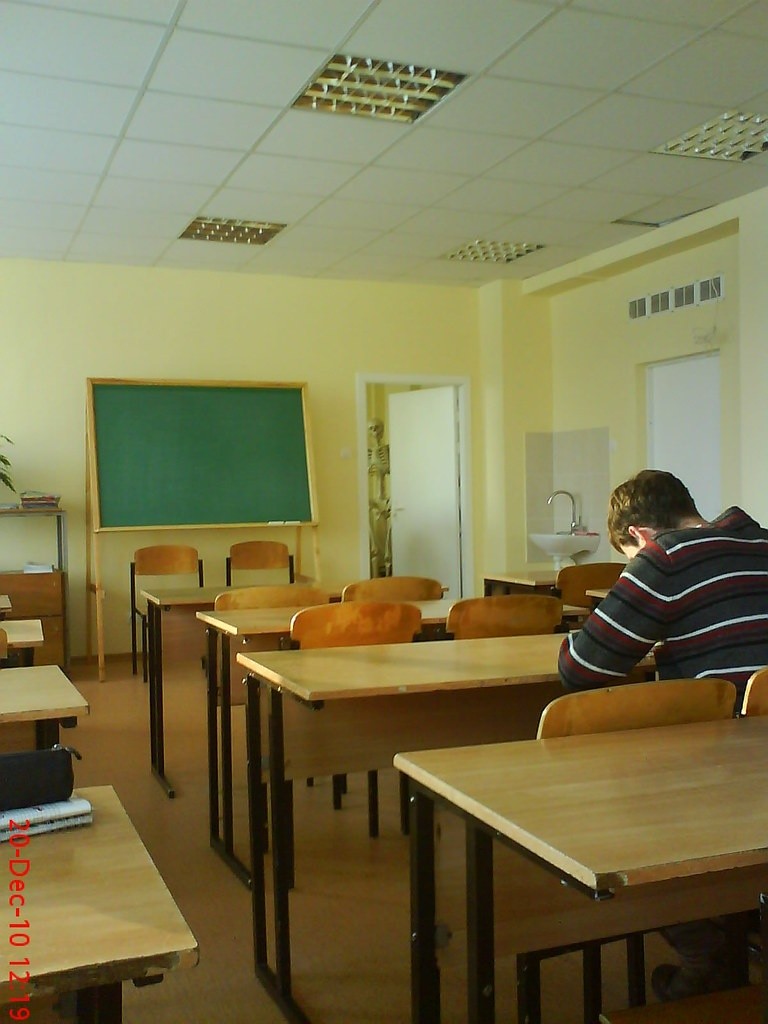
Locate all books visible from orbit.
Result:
[1,811,95,845]
[24,563,55,574]
[18,490,61,510]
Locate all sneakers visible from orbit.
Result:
[652,963,750,1007]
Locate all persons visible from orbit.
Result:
[556,469,768,997]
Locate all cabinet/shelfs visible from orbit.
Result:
[0,507,68,671]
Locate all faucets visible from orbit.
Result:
[546,489,577,530]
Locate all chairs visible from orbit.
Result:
[226,538,294,587]
[290,594,768,1024]
[131,545,208,683]
[551,561,628,608]
[213,585,329,610]
[339,578,444,600]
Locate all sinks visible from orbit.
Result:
[527,532,602,557]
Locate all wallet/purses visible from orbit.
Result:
[1,745,83,811]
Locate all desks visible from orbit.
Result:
[0,594,14,620]
[585,588,612,610]
[196,598,591,888]
[0,666,89,751]
[482,568,563,597]
[235,630,675,1024]
[393,711,767,1024]
[141,582,450,798]
[0,785,200,1023]
[0,619,45,667]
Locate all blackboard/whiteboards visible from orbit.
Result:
[83,377,320,537]
